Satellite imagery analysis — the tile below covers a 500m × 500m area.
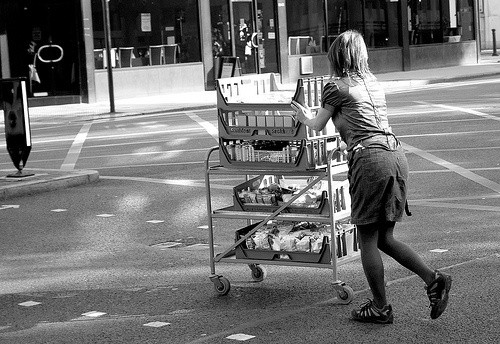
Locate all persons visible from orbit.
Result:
[290,30,453,325]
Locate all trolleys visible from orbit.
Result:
[205,146,355,304]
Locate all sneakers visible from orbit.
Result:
[424,270,451,319]
[352,297,393,325]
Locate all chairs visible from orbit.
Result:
[150,46,163,66]
[119,47,134,68]
[163,44,178,64]
[321,36,337,52]
[288,36,310,54]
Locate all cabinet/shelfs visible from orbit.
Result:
[204,144,360,305]
